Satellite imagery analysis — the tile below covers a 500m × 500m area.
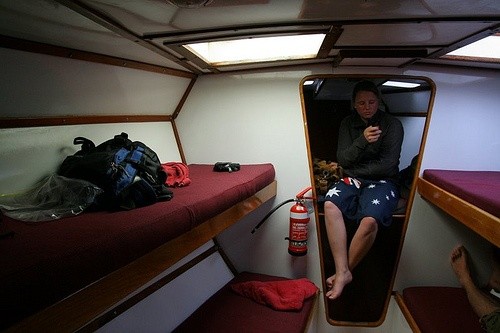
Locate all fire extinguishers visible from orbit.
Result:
[284,185,316,259]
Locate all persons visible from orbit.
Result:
[449,243,500,333]
[323,81,404,299]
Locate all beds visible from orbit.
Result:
[0,164,276,333]
[171,272,319,333]
[417,170,500,254]
[395,286,487,333]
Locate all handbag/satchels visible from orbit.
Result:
[57,133,173,208]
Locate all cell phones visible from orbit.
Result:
[367,119,379,127]
